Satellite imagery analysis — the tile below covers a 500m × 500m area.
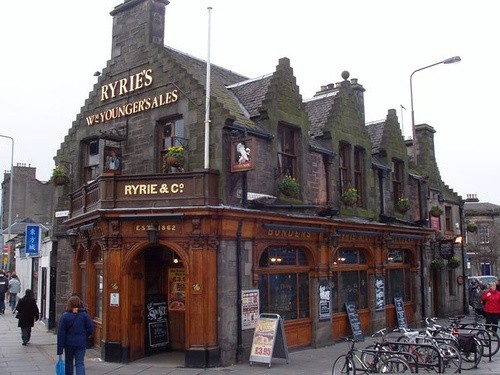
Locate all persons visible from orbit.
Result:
[0,269,21,315]
[467,278,500,337]
[16,289,40,345]
[56,292,95,375]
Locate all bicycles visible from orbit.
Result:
[332,314,500,375]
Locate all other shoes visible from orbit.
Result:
[22,343,27,345]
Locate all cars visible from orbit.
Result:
[467,276,497,304]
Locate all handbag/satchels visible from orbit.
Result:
[56,355,65,375]
[15,313,19,319]
[454,334,477,352]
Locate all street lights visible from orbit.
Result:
[446,197,480,315]
[410,55,461,164]
[0,135,14,273]
